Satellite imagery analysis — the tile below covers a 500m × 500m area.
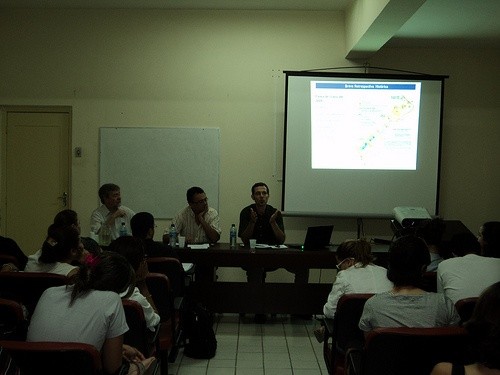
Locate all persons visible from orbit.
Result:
[164,187,222,243]
[89,183,136,246]
[360,235,463,375]
[27,251,157,375]
[0,209,211,333]
[408,216,500,305]
[314,239,393,342]
[238,182,286,324]
[431,281,500,375]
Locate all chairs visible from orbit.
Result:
[314,293,481,375]
[0,257,196,375]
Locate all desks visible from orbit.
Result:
[166,243,392,318]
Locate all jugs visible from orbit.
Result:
[101,225,112,245]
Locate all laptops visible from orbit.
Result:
[284,224,335,250]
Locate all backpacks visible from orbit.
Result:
[184,308,217,359]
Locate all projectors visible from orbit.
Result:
[392,206,432,228]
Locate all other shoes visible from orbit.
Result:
[313,326,327,343]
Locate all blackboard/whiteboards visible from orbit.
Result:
[100,127,220,221]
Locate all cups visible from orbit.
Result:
[250,240,256,248]
[179,236,185,248]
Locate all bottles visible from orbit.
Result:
[170,224,176,248]
[120,222,127,237]
[230,224,237,247]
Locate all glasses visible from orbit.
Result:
[193,198,208,205]
[336,258,355,270]
